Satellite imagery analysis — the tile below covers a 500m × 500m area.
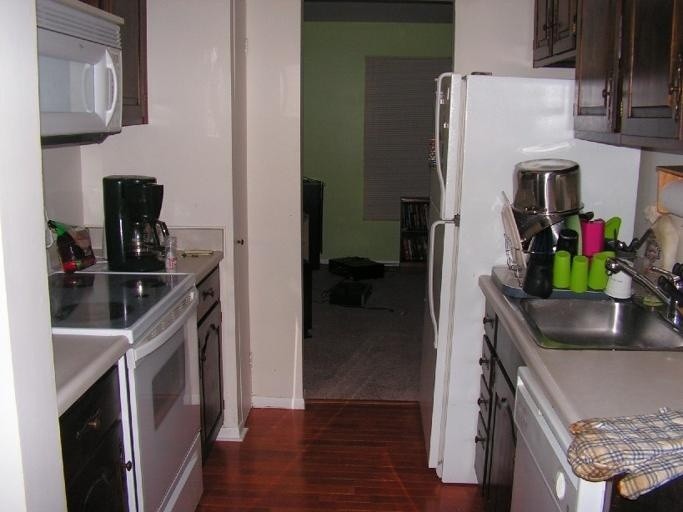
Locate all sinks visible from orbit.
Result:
[507,285,683,353]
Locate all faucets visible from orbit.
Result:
[604,256,683,336]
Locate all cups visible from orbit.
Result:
[552,251,570,289]
[522,255,553,299]
[588,254,608,291]
[569,256,589,294]
[164,236,178,271]
[556,229,582,254]
[604,258,633,299]
[601,251,617,262]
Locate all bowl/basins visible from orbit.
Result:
[510,157,585,215]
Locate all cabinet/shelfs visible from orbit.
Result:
[571,0,683,155]
[466,274,527,512]
[81,235,226,465]
[510,362,606,512]
[55,337,136,512]
[532,0,578,70]
[36,1,125,147]
[47,271,207,512]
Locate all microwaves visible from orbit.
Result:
[38,24,124,146]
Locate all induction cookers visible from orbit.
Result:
[47,274,199,335]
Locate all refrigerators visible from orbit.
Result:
[417,73,644,485]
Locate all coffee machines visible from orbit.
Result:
[102,173,169,271]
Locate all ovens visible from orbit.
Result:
[125,286,203,512]
